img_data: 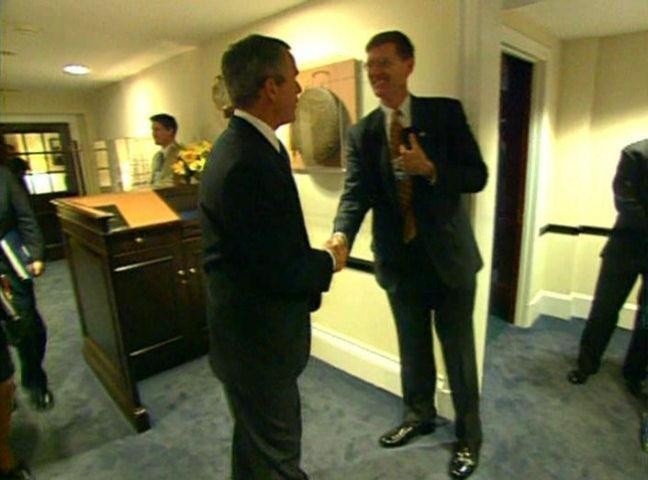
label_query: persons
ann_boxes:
[567,138,648,398]
[329,30,488,479]
[149,113,186,191]
[0,157,53,479]
[200,34,348,480]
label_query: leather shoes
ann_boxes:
[449,448,479,480]
[381,425,435,448]
[632,379,647,401]
[31,390,53,411]
[569,364,586,384]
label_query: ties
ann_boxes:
[389,110,417,244]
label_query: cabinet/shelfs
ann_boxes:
[50,187,210,434]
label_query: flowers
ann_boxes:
[171,137,213,181]
